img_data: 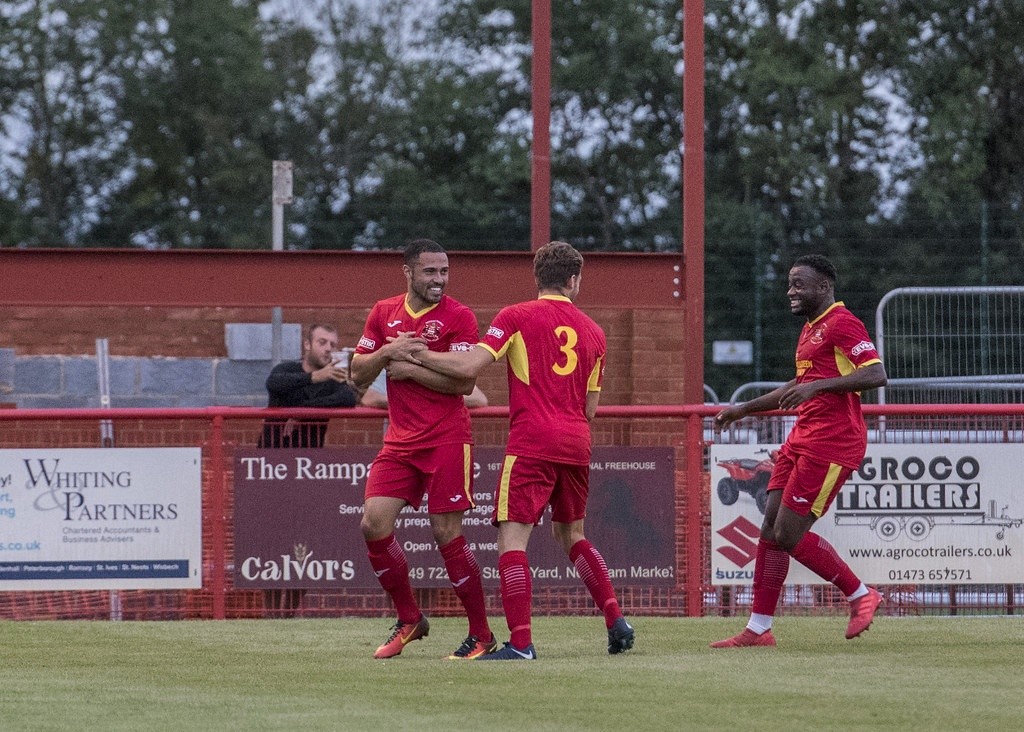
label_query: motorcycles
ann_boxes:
[715,448,784,514]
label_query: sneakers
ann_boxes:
[475,642,536,660]
[444,632,497,660]
[374,611,430,658]
[607,617,636,654]
[709,628,776,647]
[846,586,884,639]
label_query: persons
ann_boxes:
[707,254,888,649]
[257,320,359,616]
[363,368,487,619]
[350,239,501,661]
[384,239,636,661]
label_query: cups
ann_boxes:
[342,348,356,379]
[331,352,349,379]
